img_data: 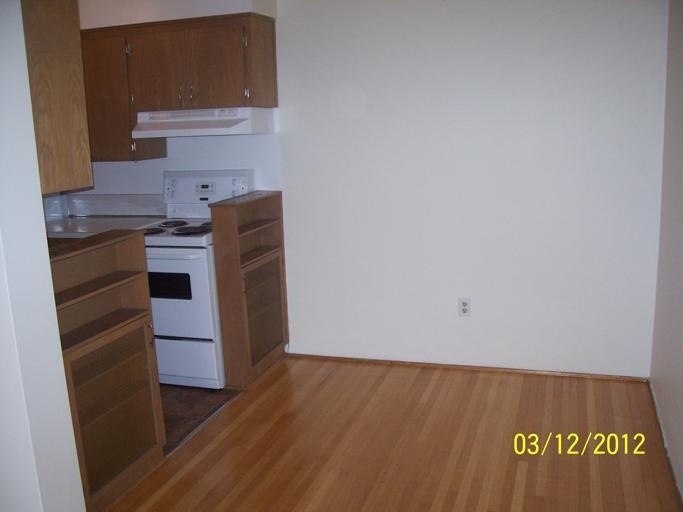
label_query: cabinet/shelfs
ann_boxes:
[125,13,278,112]
[49,228,166,511]
[208,191,289,389]
[19,1,96,199]
[80,26,167,163]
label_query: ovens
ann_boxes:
[145,245,216,341]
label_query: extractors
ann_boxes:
[131,107,272,140]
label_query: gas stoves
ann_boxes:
[130,218,212,243]
